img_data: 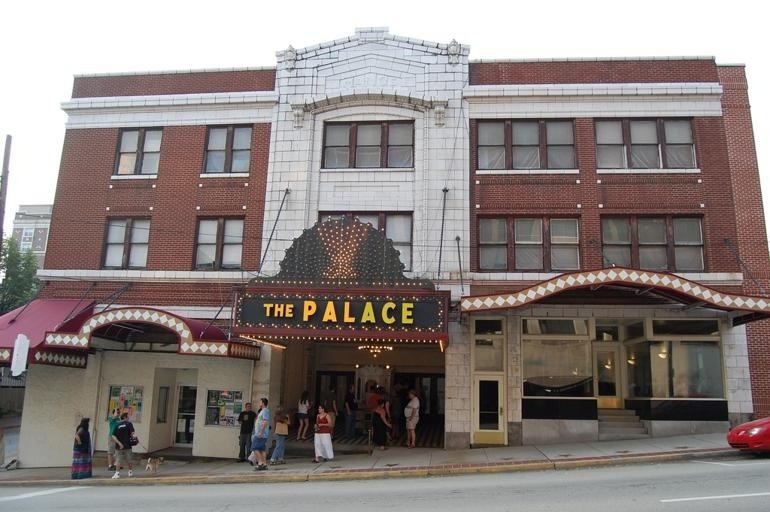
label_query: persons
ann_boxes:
[237,379,422,471]
[107,408,124,470]
[111,412,136,479]
[70,417,92,478]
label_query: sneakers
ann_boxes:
[108,465,132,479]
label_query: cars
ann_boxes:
[650,393,729,419]
[727,416,770,457]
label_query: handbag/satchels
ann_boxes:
[275,422,288,436]
[130,436,138,446]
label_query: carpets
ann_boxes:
[285,409,445,449]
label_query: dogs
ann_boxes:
[144,456,164,472]
